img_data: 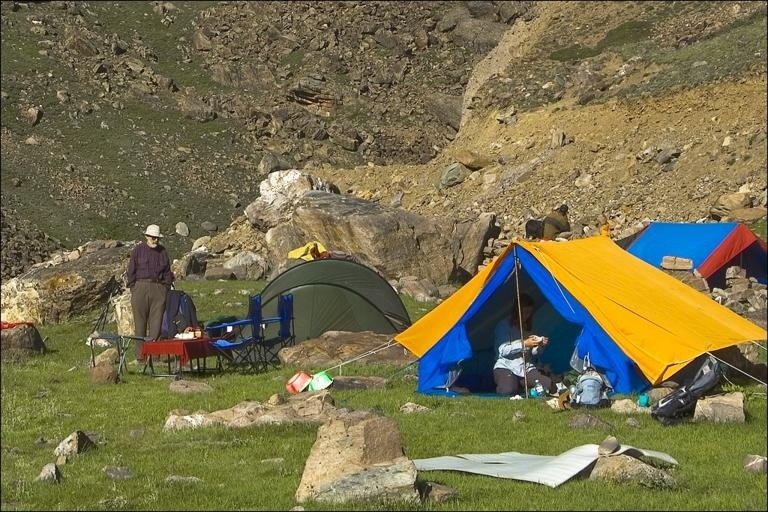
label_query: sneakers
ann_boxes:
[136,358,164,367]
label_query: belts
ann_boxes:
[134,278,164,284]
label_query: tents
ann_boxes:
[228,258,412,368]
[394,235,767,417]
[611,221,768,291]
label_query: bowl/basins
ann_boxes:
[306,372,335,392]
[285,371,314,395]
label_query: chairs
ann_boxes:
[88,290,296,380]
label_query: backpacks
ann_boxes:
[571,367,610,408]
[648,389,697,423]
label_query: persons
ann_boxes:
[541,203,571,241]
[492,292,551,397]
[126,223,172,368]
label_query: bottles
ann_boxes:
[534,379,546,398]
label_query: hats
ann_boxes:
[142,224,165,239]
